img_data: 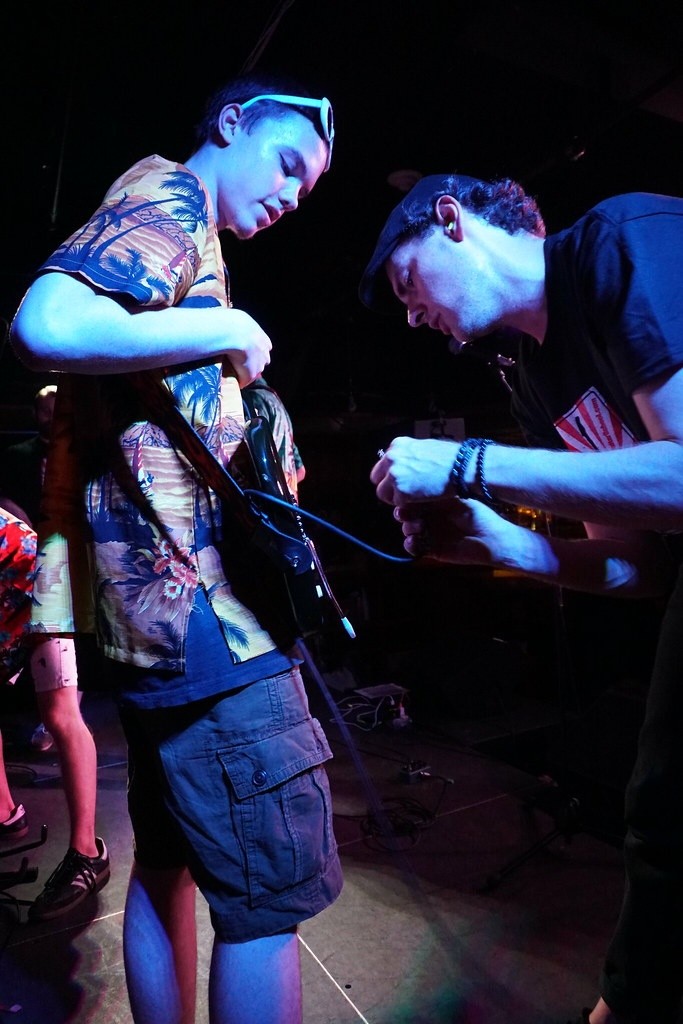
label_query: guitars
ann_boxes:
[215,254,358,654]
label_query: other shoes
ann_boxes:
[32,725,53,750]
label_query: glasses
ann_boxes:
[240,94,336,174]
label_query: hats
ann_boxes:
[360,173,482,312]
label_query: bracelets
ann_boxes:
[449,437,497,505]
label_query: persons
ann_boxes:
[357,172,682,1023]
[0,77,345,1024]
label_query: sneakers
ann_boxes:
[28,835,110,920]
[0,804,30,839]
